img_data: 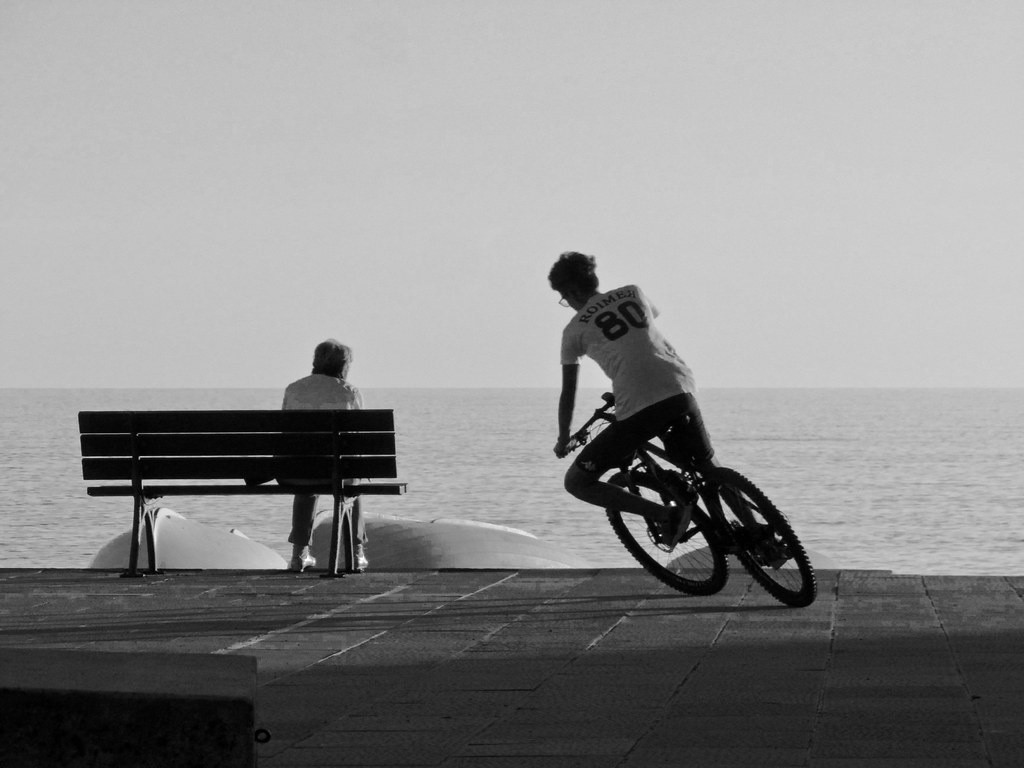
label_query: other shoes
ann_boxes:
[354,554,369,569]
[291,554,316,572]
[663,506,691,547]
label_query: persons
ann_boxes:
[548,251,792,570]
[275,338,371,573]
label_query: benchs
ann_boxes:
[77,408,409,581]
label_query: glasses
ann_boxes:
[559,294,569,307]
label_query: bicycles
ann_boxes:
[564,391,818,609]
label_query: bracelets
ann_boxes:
[558,434,571,447]
